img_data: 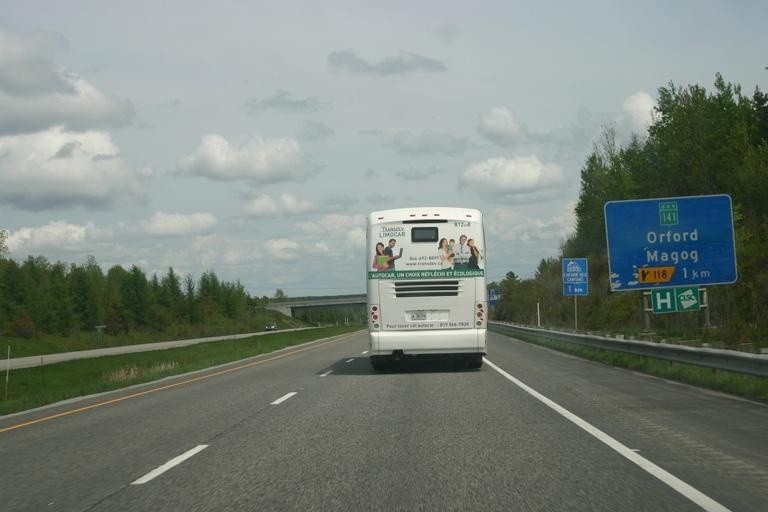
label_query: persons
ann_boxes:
[384,239,403,269]
[372,241,389,271]
[437,235,480,270]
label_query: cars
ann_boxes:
[265,322,277,330]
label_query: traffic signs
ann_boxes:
[607,194,735,288]
[563,258,587,296]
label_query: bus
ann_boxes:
[362,205,490,369]
